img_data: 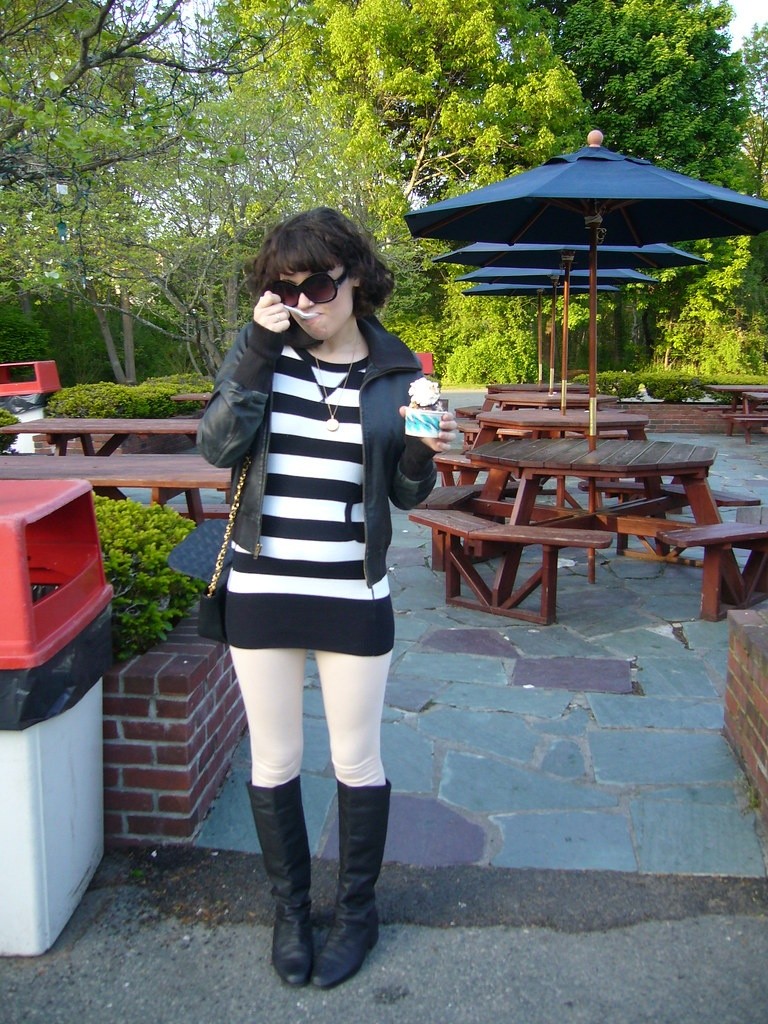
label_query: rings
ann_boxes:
[275,314,280,323]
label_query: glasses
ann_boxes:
[264,263,349,308]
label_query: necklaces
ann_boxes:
[315,328,359,431]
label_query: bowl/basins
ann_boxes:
[405,407,446,438]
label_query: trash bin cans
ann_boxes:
[0,359,62,454]
[412,353,434,378]
[2,477,114,954]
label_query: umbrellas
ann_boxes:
[404,130,768,452]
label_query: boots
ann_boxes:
[246,775,311,987]
[309,779,392,991]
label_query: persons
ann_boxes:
[196,207,456,989]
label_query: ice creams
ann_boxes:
[404,377,444,439]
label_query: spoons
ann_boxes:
[260,297,319,319]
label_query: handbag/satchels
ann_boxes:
[167,518,232,643]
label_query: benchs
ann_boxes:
[407,404,768,625]
[142,502,231,519]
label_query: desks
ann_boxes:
[473,410,649,432]
[484,392,619,407]
[464,439,745,607]
[171,393,211,408]
[704,385,767,391]
[486,384,593,392]
[0,452,232,526]
[0,418,201,456]
[742,392,768,402]
